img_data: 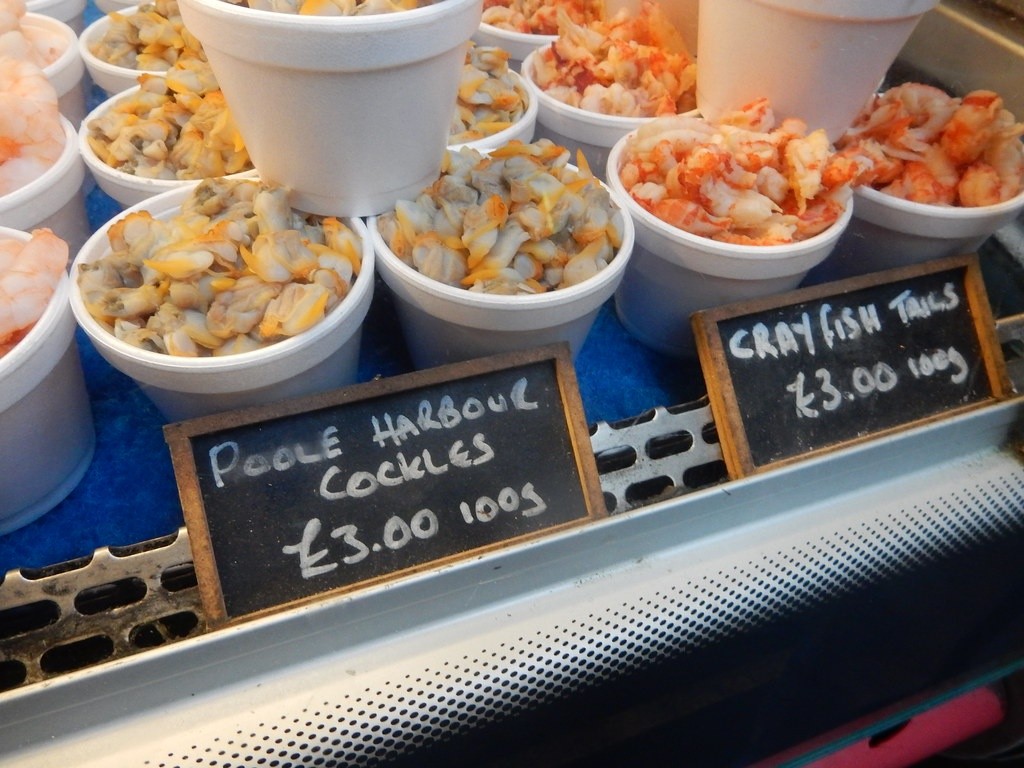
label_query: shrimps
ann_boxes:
[619,96,844,245]
[1,1,69,357]
[823,82,1024,210]
[480,0,697,117]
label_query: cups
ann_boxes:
[0,0,1024,535]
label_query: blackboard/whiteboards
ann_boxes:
[690,254,1016,482]
[162,338,608,633]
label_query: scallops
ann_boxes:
[77,176,363,358]
[89,0,210,73]
[87,59,254,179]
[377,137,622,297]
[221,0,434,17]
[445,39,527,145]
